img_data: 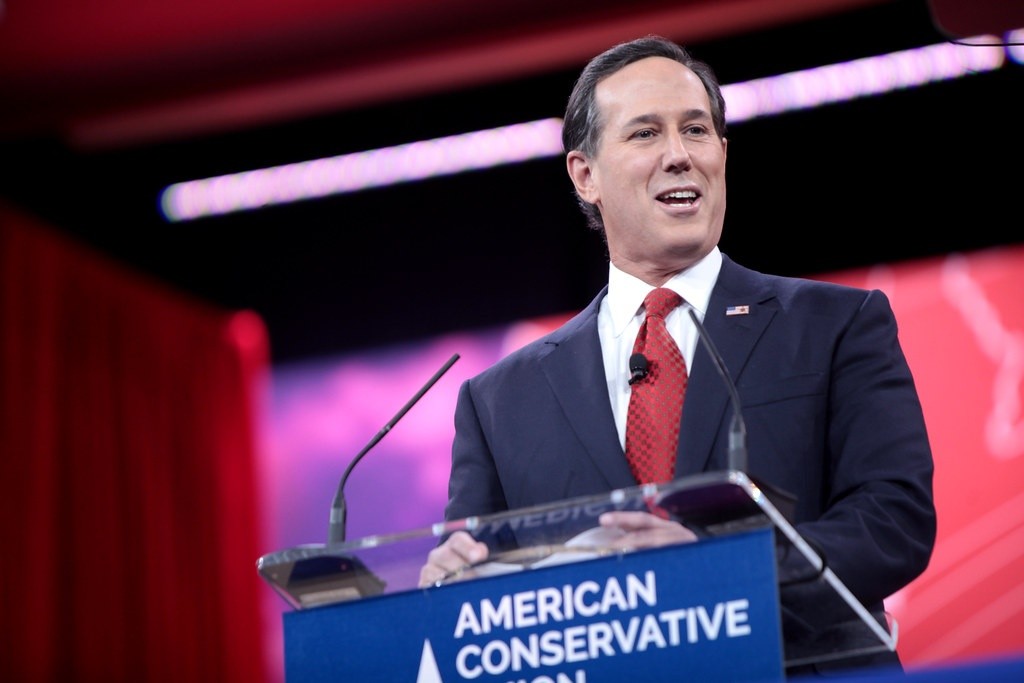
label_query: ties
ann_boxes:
[624,288,689,521]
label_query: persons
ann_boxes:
[417,34,938,679]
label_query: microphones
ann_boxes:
[287,353,461,608]
[630,353,647,379]
[657,309,798,538]
